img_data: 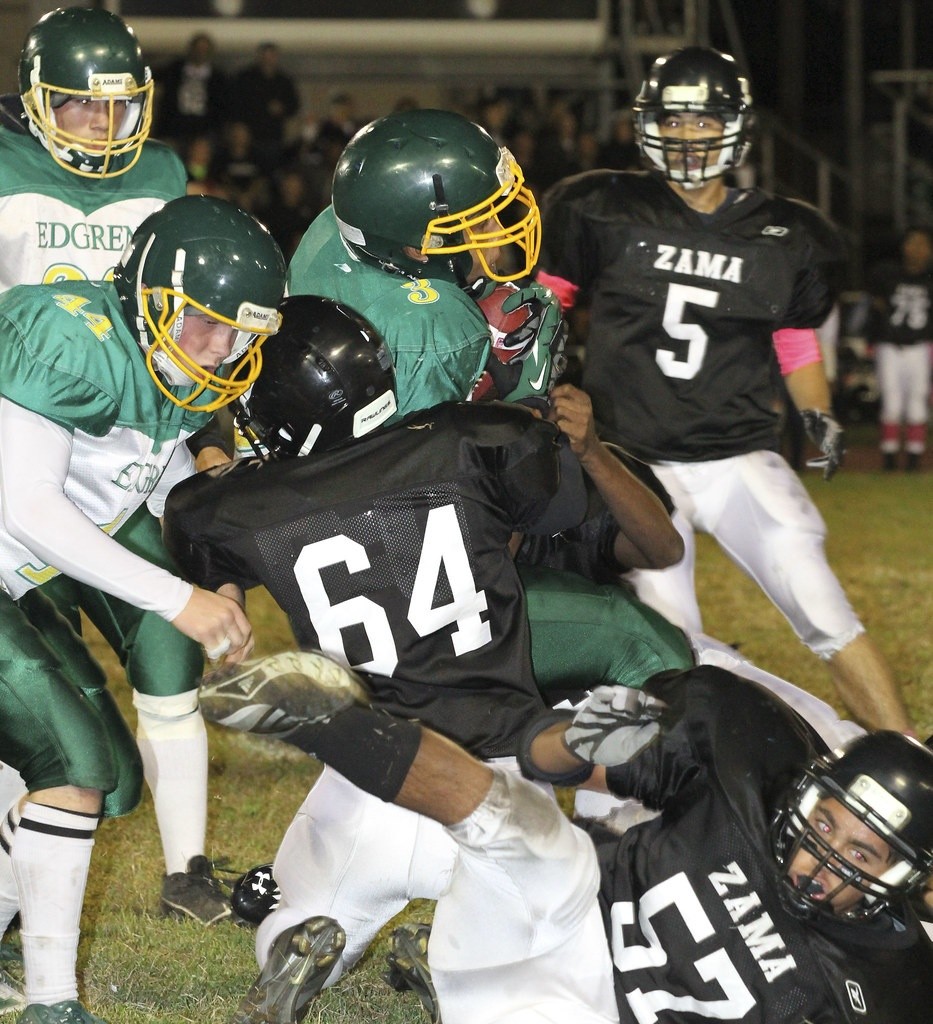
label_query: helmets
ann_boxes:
[228,296,399,457]
[763,727,933,930]
[15,7,157,179]
[112,192,288,412]
[332,109,541,299]
[632,46,758,189]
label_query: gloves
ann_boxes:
[561,685,674,768]
[484,284,569,419]
[802,408,848,482]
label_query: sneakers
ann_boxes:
[229,860,282,923]
[159,854,234,926]
[17,1000,108,1024]
[198,649,391,744]
[383,921,442,1024]
[0,939,28,1002]
[227,914,347,1024]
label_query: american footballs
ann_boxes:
[464,283,536,403]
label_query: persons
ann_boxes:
[0,7,933,1024]
[529,46,915,741]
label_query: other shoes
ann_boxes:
[881,449,896,472]
[906,451,923,473]
